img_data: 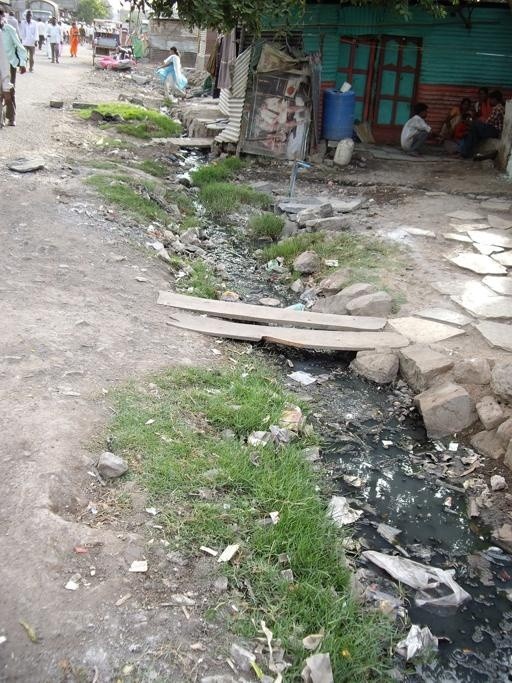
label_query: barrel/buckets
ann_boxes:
[322,89,356,139]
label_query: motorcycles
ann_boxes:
[117,46,134,61]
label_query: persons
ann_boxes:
[37,17,119,49]
[0,5,29,129]
[400,101,433,158]
[19,8,39,73]
[440,86,505,156]
[157,46,189,102]
[47,16,61,63]
[69,22,79,57]
